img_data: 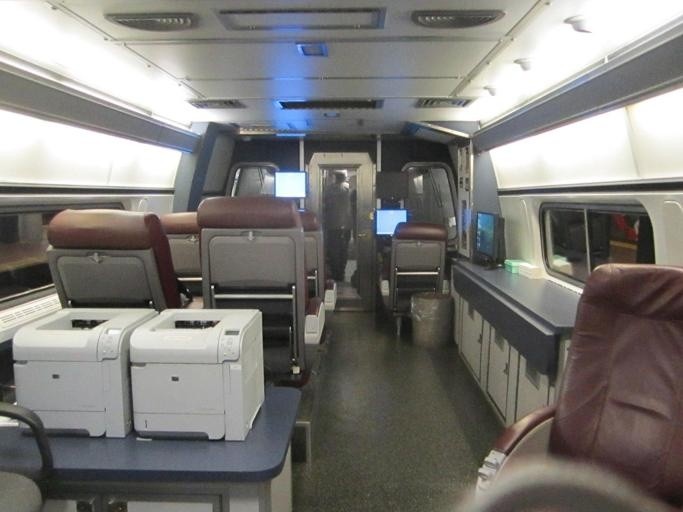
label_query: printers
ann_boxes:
[127,307,266,442]
[11,307,160,438]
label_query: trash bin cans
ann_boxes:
[411,291,453,350]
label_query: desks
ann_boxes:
[0,386,303,511]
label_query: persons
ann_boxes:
[324,169,358,282]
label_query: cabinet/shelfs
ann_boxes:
[450,258,582,429]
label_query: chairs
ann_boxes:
[161,211,202,291]
[0,404,53,512]
[476,262,683,512]
[379,222,447,335]
[46,208,181,309]
[198,196,306,371]
[298,211,325,301]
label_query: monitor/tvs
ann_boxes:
[474,209,506,270]
[374,208,409,237]
[274,171,308,198]
